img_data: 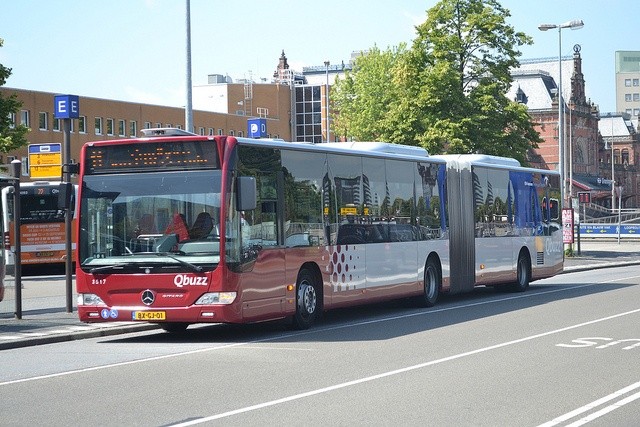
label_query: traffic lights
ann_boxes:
[577,192,591,205]
[542,196,560,222]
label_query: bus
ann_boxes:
[0,180,79,276]
[57,127,564,333]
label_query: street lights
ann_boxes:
[538,19,584,211]
